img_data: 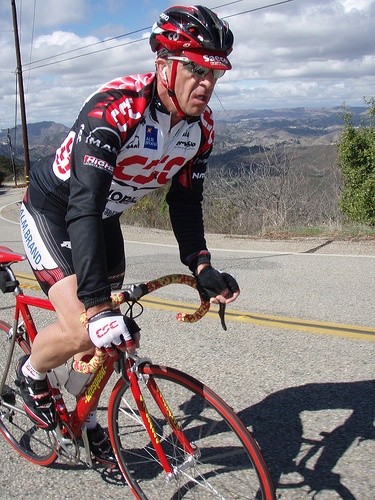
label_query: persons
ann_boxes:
[15,4,240,464]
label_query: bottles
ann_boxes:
[64,354,97,398]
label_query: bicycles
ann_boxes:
[0,245,277,500]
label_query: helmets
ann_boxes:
[149,4,234,70]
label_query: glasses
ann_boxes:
[165,56,226,78]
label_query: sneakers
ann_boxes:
[73,423,117,463]
[15,354,58,430]
